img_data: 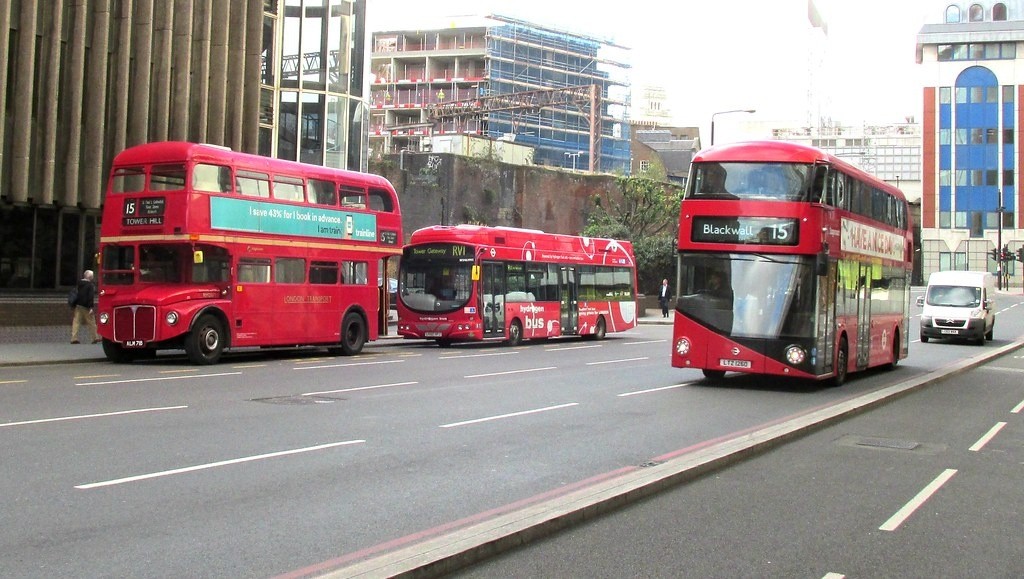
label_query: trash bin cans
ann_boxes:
[638,293,646,317]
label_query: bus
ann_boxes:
[96,142,402,366]
[671,141,913,386]
[396,224,639,348]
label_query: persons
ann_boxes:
[70,270,102,344]
[385,92,391,104]
[439,89,445,103]
[657,278,672,318]
[706,273,723,289]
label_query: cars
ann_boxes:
[378,276,403,304]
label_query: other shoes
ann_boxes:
[71,340,80,344]
[92,337,102,344]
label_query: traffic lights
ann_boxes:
[1016,247,1024,263]
[990,248,997,261]
[1000,247,1015,261]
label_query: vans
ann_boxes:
[915,270,996,345]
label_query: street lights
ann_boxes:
[563,150,584,170]
[710,108,757,146]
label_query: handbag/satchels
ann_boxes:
[68,285,78,306]
